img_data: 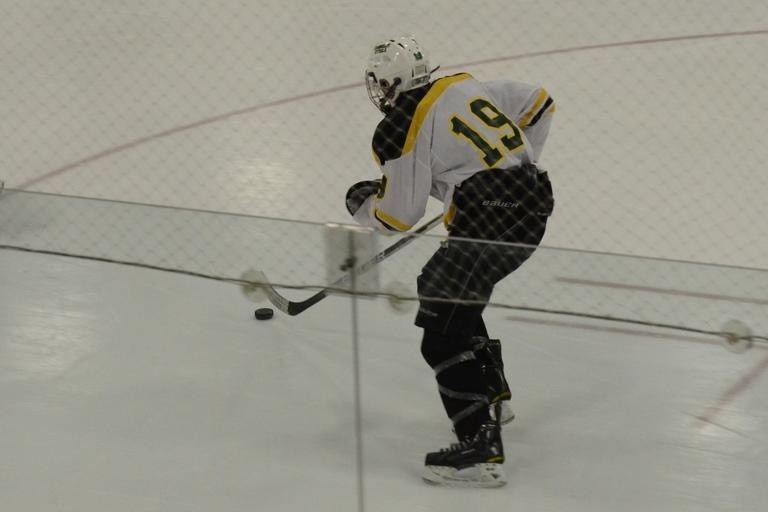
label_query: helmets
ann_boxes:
[364,37,432,94]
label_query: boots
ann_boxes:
[463,335,514,403]
[423,422,505,468]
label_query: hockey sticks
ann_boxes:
[260,213,445,316]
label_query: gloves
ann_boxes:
[344,176,383,217]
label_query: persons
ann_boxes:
[345,34,556,473]
